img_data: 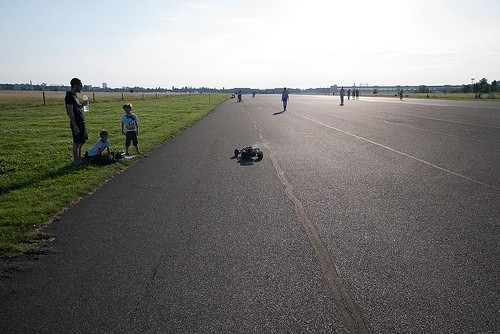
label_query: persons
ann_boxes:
[235,91,237,95]
[340,86,360,106]
[237,90,242,102]
[121,103,143,156]
[84,130,110,163]
[252,92,255,98]
[399,89,404,99]
[281,87,289,111]
[65,78,89,166]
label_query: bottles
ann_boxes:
[82,95,90,112]
